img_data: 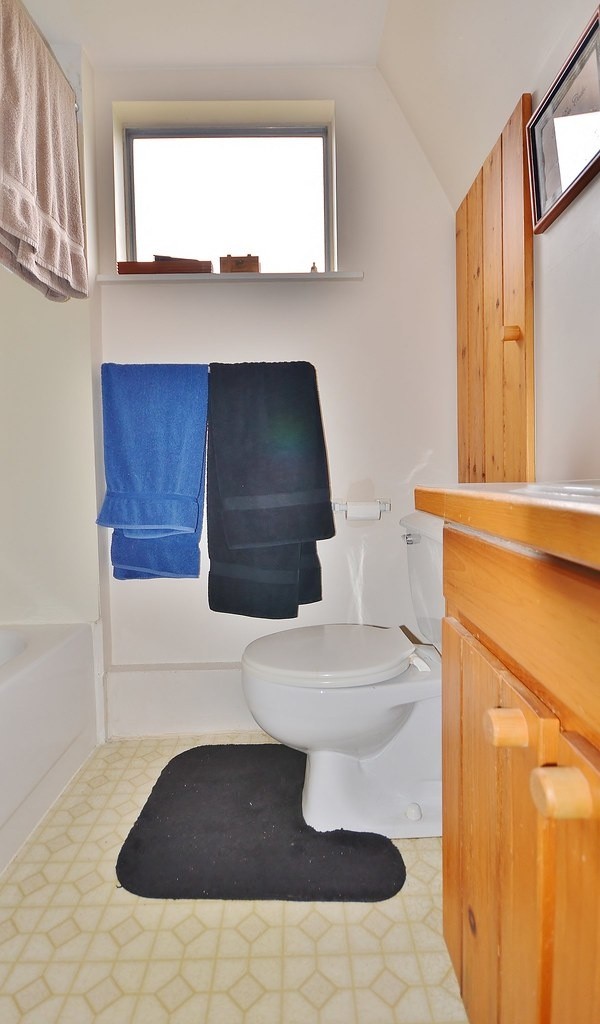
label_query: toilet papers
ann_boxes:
[345,500,381,521]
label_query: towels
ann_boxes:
[208,361,341,620]
[95,362,209,582]
[1,0,90,303]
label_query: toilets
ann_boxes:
[241,510,443,839]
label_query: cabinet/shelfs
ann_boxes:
[413,3,600,1024]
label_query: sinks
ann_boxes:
[507,478,600,503]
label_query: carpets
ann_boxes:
[115,743,407,904]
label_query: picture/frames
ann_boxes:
[525,6,600,235]
